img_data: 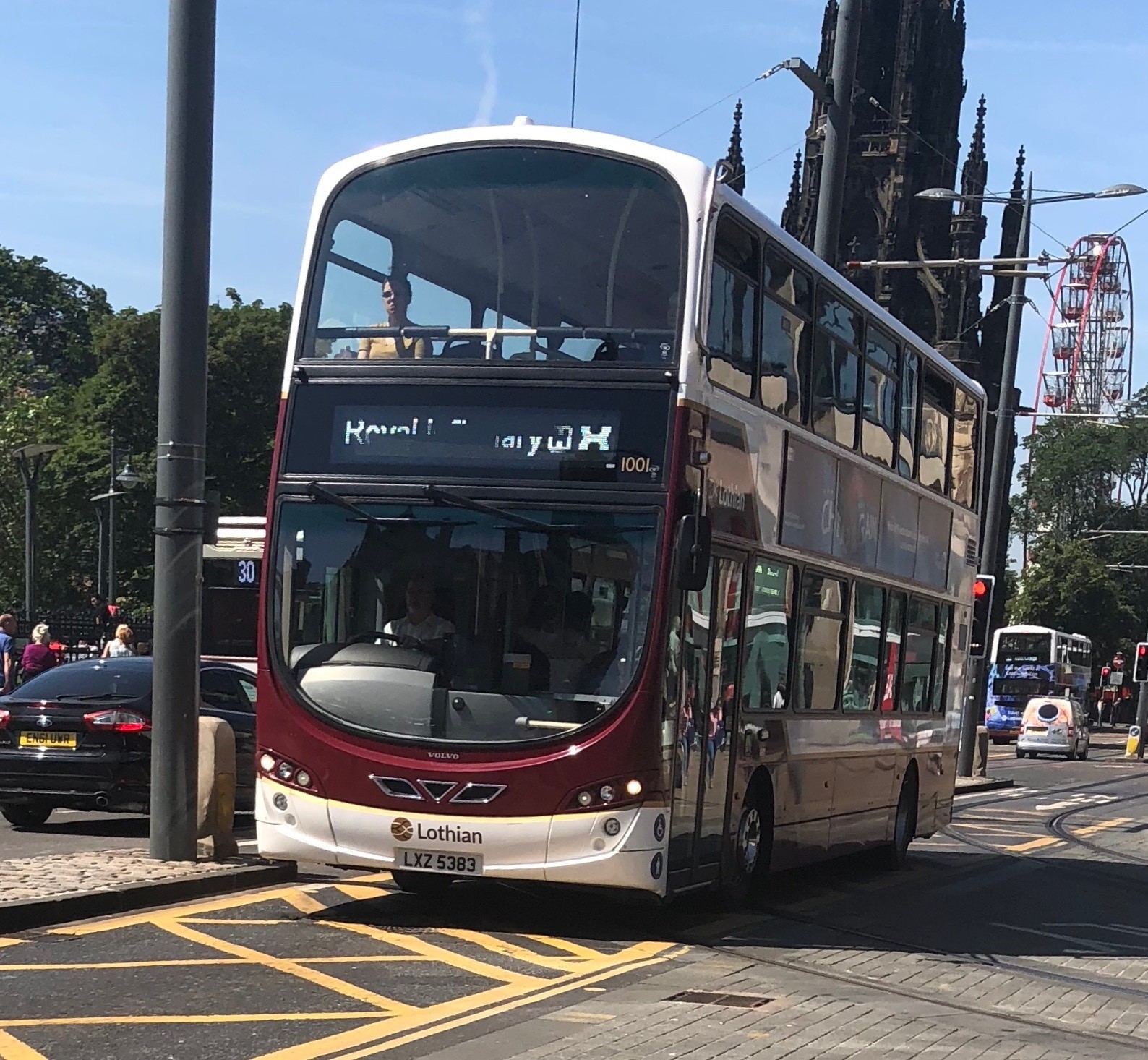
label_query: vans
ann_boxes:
[1015,698,1093,760]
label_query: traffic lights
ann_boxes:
[1100,667,1111,686]
[969,574,996,659]
[1132,642,1148,682]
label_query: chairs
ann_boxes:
[440,336,502,360]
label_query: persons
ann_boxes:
[88,590,121,621]
[0,614,17,698]
[98,624,138,659]
[372,570,457,669]
[18,621,57,685]
[705,694,730,791]
[356,273,438,361]
[771,682,786,710]
[675,696,695,783]
[669,612,681,715]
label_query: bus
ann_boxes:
[254,117,987,910]
[985,624,1092,744]
[201,516,351,711]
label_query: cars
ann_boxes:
[0,657,260,827]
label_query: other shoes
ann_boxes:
[709,781,713,788]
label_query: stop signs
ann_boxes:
[1112,655,1125,668]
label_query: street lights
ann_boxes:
[109,445,146,606]
[914,184,1148,779]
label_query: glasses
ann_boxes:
[383,291,408,298]
